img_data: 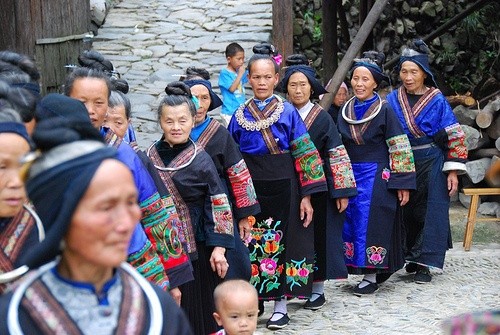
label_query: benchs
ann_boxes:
[462,188,500,252]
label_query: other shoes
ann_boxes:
[353,279,380,296]
[255,304,290,328]
[304,292,327,310]
[406,261,432,284]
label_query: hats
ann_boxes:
[349,62,392,86]
[22,147,118,269]
[183,80,223,112]
[282,66,330,95]
[318,80,349,100]
[398,53,439,90]
[0,122,32,150]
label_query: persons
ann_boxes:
[60,50,195,309]
[209,278,260,335]
[0,50,139,147]
[386,39,469,286]
[177,63,261,284]
[100,75,194,274]
[217,42,251,128]
[0,82,46,298]
[29,118,170,296]
[224,42,329,329]
[318,78,350,125]
[0,118,195,335]
[337,49,419,297]
[274,53,358,310]
[142,81,238,335]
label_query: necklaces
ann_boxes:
[341,90,382,124]
[1,204,49,286]
[7,253,164,335]
[147,135,196,172]
[233,94,287,131]
